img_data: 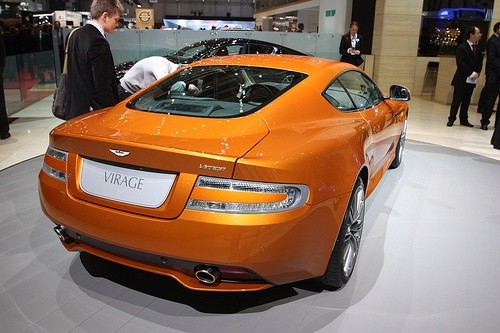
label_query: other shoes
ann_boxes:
[481,125,487,130]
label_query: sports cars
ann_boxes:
[38,54,410,293]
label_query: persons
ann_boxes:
[0,40,12,140]
[480,30,500,131]
[52,0,124,130]
[116,56,186,103]
[297,23,305,33]
[477,22,500,114]
[447,27,481,127]
[338,22,365,67]
[0,20,65,81]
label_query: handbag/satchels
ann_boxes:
[52,72,90,119]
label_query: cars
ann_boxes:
[114,38,314,81]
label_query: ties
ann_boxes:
[472,44,477,57]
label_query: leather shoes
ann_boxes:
[447,120,454,126]
[460,120,474,127]
